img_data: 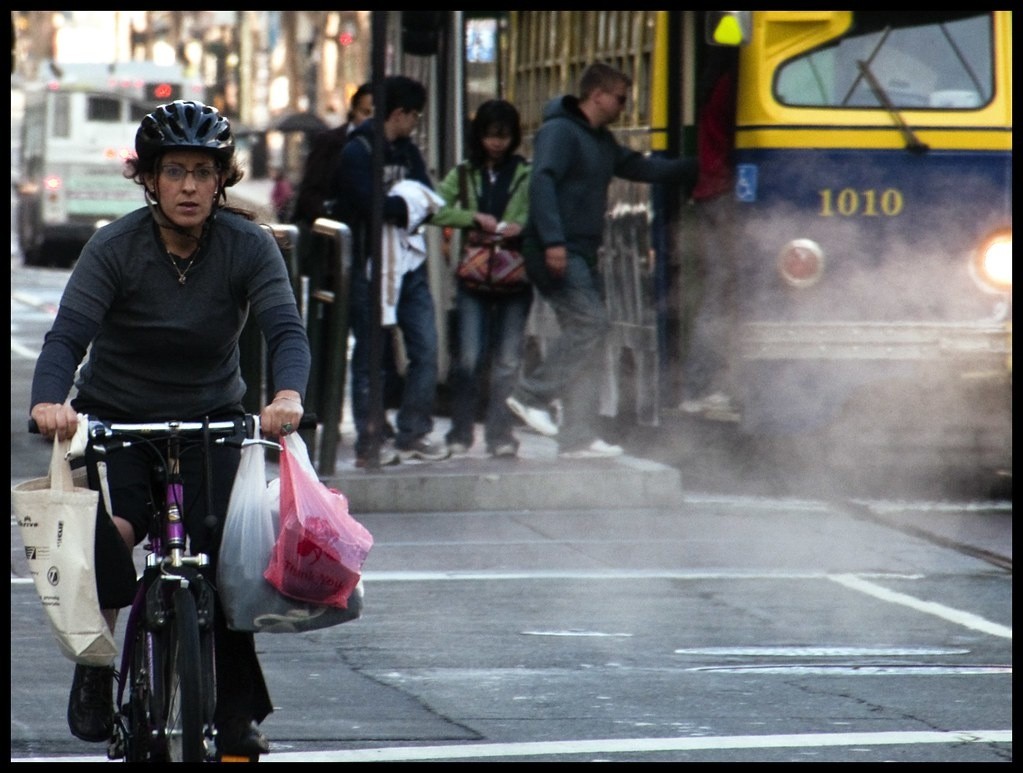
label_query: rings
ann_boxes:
[282,421,294,433]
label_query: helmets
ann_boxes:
[135,99,236,159]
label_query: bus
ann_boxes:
[13,60,199,268]
[350,9,1013,491]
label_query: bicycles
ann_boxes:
[28,410,318,763]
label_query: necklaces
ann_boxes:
[165,244,201,283]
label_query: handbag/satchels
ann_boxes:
[12,413,120,662]
[455,230,533,298]
[215,419,375,633]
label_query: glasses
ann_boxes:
[157,163,220,182]
[603,89,627,106]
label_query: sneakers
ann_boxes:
[355,438,452,466]
[66,661,114,742]
[216,715,270,754]
[560,439,625,459]
[506,396,560,436]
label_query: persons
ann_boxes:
[29,99,309,754]
[272,74,535,472]
[507,61,683,460]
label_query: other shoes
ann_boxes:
[447,441,515,461]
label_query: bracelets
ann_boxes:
[273,396,302,405]
